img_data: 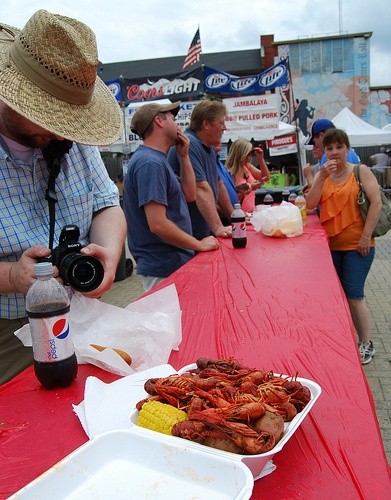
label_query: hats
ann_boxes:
[1,9,124,146]
[307,118,334,145]
[130,99,181,137]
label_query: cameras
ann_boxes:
[36,224,105,293]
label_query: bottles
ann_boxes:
[282,187,290,201]
[230,203,247,249]
[288,189,297,204]
[23,262,78,390]
[295,191,307,226]
[263,191,274,206]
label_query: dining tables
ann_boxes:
[0,208,391,500]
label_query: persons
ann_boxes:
[370,147,390,187]
[303,118,360,185]
[0,9,128,389]
[123,100,220,292]
[225,138,270,212]
[215,142,252,207]
[305,129,383,363]
[115,174,125,197]
[166,98,234,240]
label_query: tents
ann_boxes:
[278,107,391,167]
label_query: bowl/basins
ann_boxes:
[6,361,323,500]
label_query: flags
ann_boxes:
[183,29,202,71]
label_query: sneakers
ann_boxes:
[357,340,376,364]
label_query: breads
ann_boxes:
[91,344,131,366]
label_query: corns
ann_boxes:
[137,399,189,436]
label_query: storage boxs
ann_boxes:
[254,186,304,204]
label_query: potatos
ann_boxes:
[201,410,284,456]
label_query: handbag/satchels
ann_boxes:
[251,198,303,237]
[353,164,391,236]
